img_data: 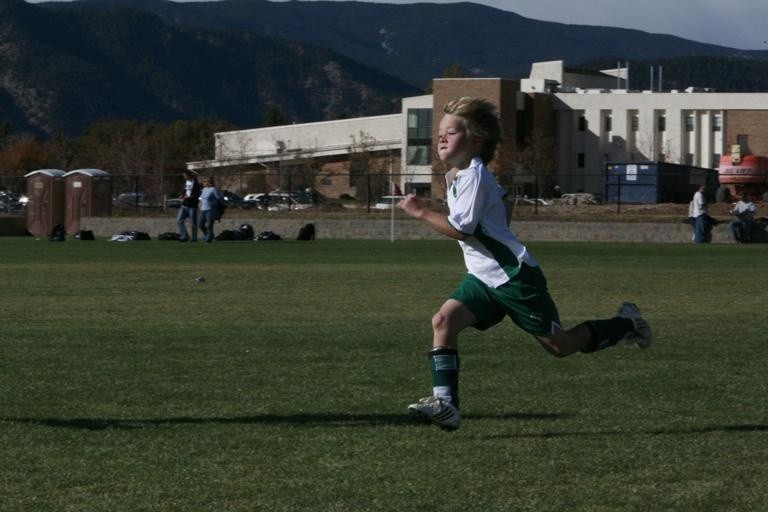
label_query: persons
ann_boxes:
[196,176,221,244]
[728,190,757,245]
[691,184,707,243]
[174,169,201,243]
[393,95,652,433]
[687,198,704,242]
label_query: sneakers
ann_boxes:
[618,302,651,350]
[407,394,460,433]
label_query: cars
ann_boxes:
[515,193,596,206]
[0,191,28,211]
[111,190,316,211]
[376,196,406,210]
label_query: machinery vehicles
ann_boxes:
[715,145,768,204]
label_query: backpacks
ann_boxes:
[48,224,180,242]
[216,223,315,242]
